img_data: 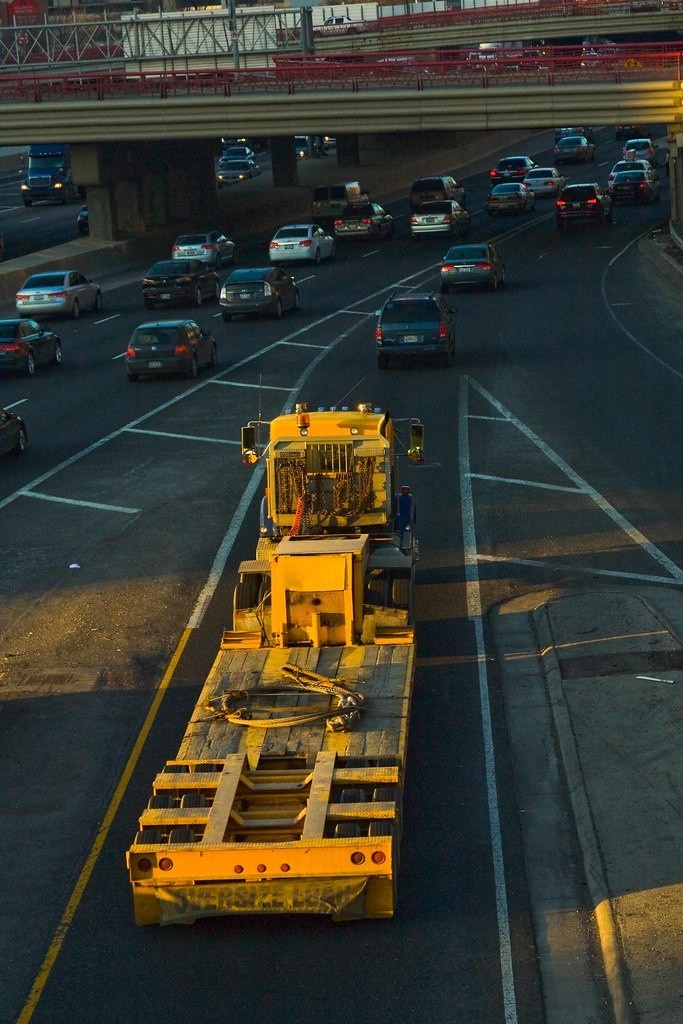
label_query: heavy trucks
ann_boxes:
[125,404,425,922]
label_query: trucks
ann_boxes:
[18,142,87,206]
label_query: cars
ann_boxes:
[486,155,565,216]
[377,42,621,71]
[312,15,368,37]
[607,149,661,203]
[219,266,299,322]
[170,229,237,267]
[554,126,660,166]
[15,271,103,320]
[269,223,337,265]
[334,201,395,243]
[78,208,91,235]
[440,243,507,291]
[0,408,27,455]
[124,318,217,383]
[0,318,63,376]
[411,201,471,240]
[218,137,335,187]
[142,260,221,312]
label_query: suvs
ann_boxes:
[373,292,459,371]
[408,175,467,209]
[309,182,371,222]
[555,183,614,227]
